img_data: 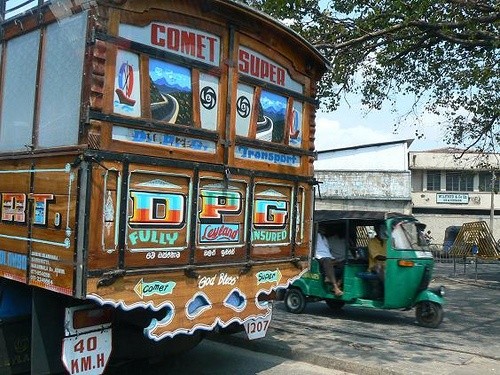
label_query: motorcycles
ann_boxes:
[275,207,446,328]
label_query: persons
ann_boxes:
[315,224,344,296]
[368,223,387,280]
[425,230,435,243]
[328,224,346,285]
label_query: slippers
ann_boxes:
[333,289,343,296]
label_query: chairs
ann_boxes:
[355,245,383,296]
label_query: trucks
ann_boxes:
[0,1,328,375]
